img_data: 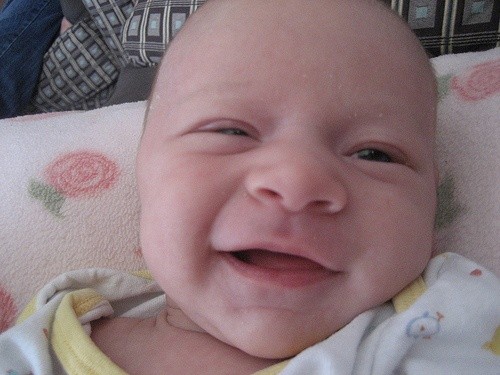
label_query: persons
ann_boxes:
[0,1,499,374]
[0,0,88,119]
